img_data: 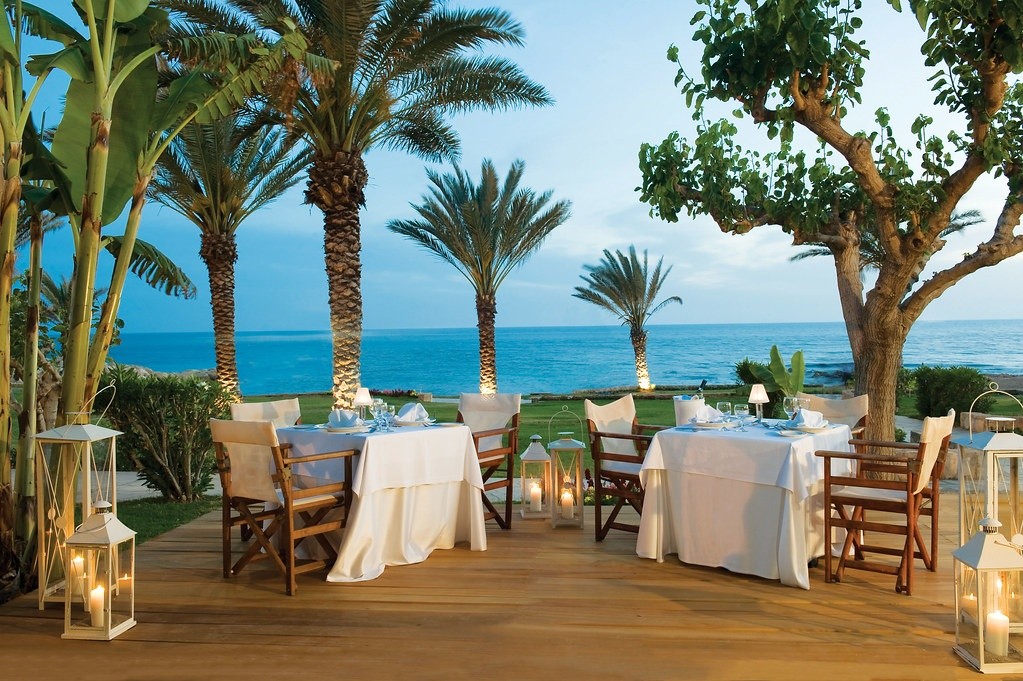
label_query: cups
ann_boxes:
[793,398,810,413]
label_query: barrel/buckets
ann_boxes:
[672,395,705,426]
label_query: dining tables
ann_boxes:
[636,416,862,594]
[258,423,488,582]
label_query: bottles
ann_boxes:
[690,380,707,400]
[396,418,437,427]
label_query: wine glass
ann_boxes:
[381,405,396,432]
[368,398,383,433]
[783,396,798,421]
[716,402,731,432]
[734,404,749,432]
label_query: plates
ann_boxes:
[776,430,808,438]
[324,426,371,433]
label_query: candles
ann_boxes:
[72,557,85,595]
[529,485,542,512]
[985,610,1011,656]
[560,491,575,518]
[997,580,1003,593]
[116,572,131,589]
[89,586,104,627]
[962,594,977,607]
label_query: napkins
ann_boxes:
[328,409,359,426]
[787,408,824,426]
[688,404,729,423]
[396,402,430,422]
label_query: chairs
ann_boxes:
[812,407,956,596]
[229,397,303,428]
[584,392,673,544]
[795,390,869,537]
[208,417,361,595]
[458,391,522,530]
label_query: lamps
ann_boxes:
[353,387,374,419]
[748,384,772,430]
